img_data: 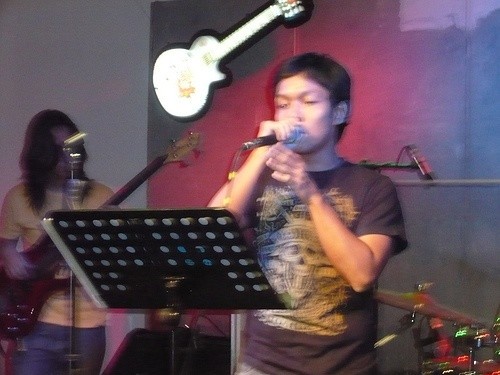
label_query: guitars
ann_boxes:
[0,131,202,339]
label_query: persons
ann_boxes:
[0,108,124,375]
[203,51,408,375]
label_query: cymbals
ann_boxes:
[375,290,475,324]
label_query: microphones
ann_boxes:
[417,284,454,358]
[242,125,305,151]
[406,145,434,181]
